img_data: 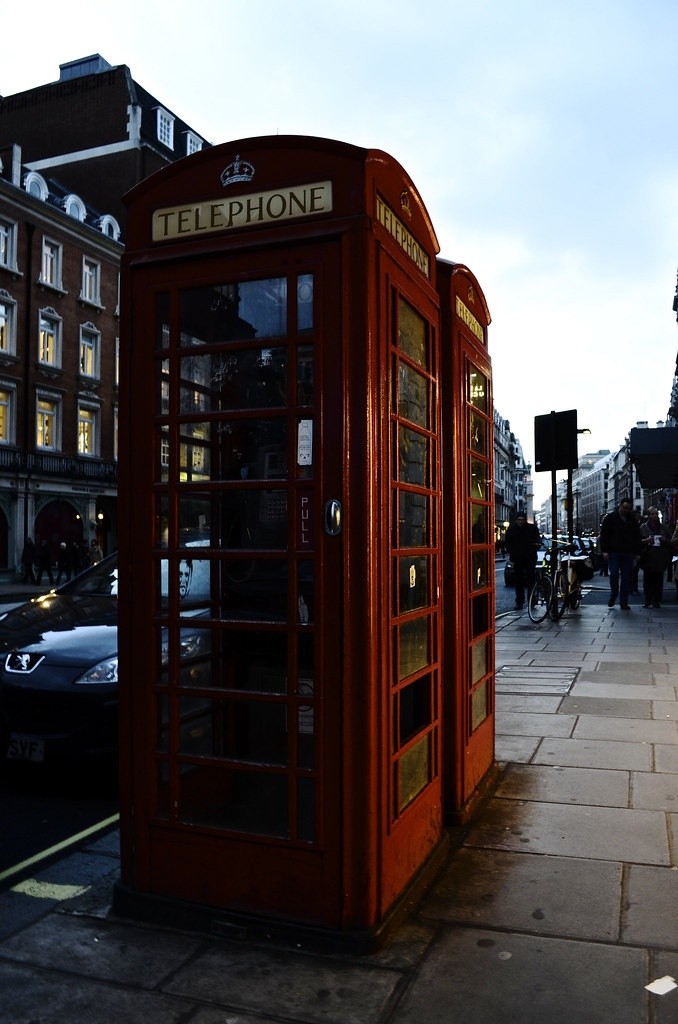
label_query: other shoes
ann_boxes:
[608,596,617,607]
[514,606,523,610]
[621,604,632,610]
[653,603,661,609]
[642,600,652,608]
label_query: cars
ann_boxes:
[504,534,599,589]
[0,528,219,770]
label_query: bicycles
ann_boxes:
[528,536,588,625]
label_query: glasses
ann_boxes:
[622,504,631,511]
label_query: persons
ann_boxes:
[640,506,672,608]
[504,511,541,610]
[600,498,641,610]
[629,505,678,599]
[21,537,104,586]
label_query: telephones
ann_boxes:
[226,442,316,546]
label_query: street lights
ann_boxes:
[573,488,583,533]
